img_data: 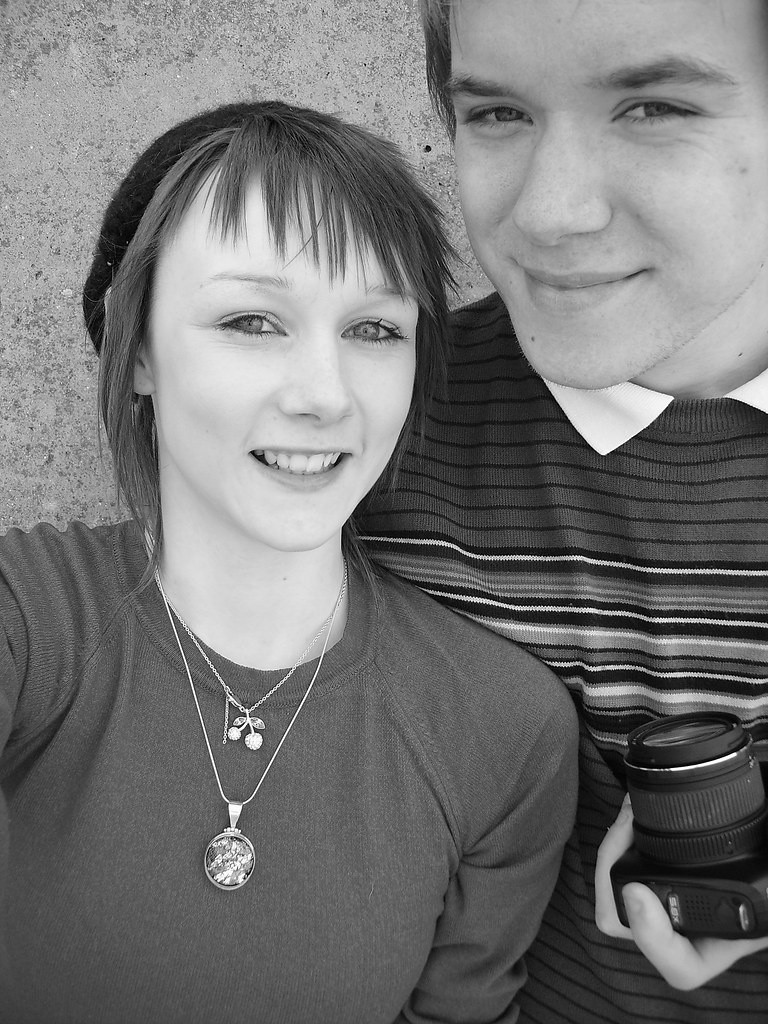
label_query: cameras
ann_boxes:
[610,710,768,940]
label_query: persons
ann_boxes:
[346,0,768,1024]
[1,102,576,1024]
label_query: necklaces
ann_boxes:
[146,528,347,893]
